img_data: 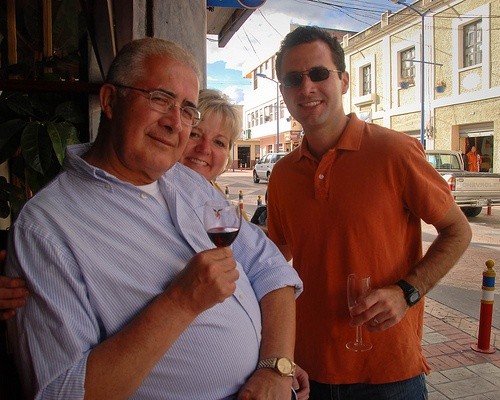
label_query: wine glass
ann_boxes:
[202,201,242,251]
[345,273,374,352]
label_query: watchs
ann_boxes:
[256,357,296,377]
[395,279,421,308]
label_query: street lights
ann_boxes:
[255,73,281,153]
[389,0,432,144]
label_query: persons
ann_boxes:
[466,146,482,173]
[0,37,309,400]
[267,25,473,400]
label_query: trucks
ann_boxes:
[424,149,500,217]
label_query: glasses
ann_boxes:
[279,66,342,88]
[112,84,204,127]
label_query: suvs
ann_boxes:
[253,152,288,183]
